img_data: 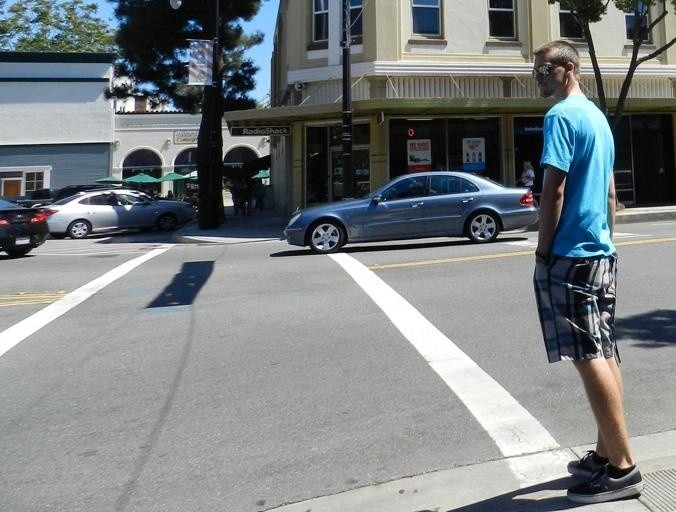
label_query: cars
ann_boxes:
[0,183,196,242]
[0,197,50,260]
[277,167,540,257]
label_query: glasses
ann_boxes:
[531,64,563,79]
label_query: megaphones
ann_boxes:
[294,80,307,91]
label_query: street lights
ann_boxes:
[166,0,224,234]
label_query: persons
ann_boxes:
[531,40,644,505]
[230,180,267,217]
[514,161,535,191]
[152,187,200,212]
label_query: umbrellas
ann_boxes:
[159,172,189,198]
[251,171,270,185]
[121,173,163,191]
[95,175,120,189]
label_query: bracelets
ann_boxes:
[534,250,550,260]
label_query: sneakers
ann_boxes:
[567,450,610,475]
[566,464,644,503]
